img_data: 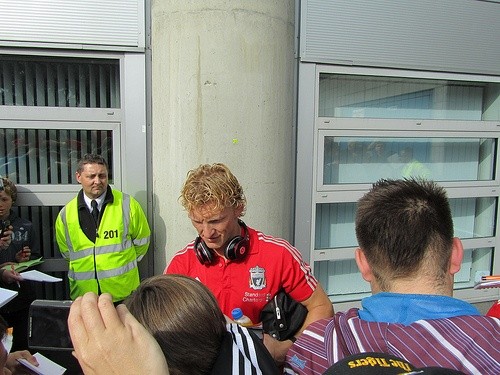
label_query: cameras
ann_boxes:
[27,299,75,351]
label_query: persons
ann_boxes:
[66,277,232,375]
[324,136,434,180]
[286,182,500,375]
[55,154,149,303]
[0,177,38,375]
[163,162,334,363]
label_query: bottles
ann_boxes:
[231,308,253,326]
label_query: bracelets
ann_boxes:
[289,336,297,343]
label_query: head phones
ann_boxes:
[194,219,249,265]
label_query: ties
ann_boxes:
[91,200,99,225]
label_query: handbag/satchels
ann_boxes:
[259,288,307,343]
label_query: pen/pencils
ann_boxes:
[11,264,21,288]
[481,275,500,281]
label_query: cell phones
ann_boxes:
[1,220,10,237]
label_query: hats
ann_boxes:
[322,352,467,375]
